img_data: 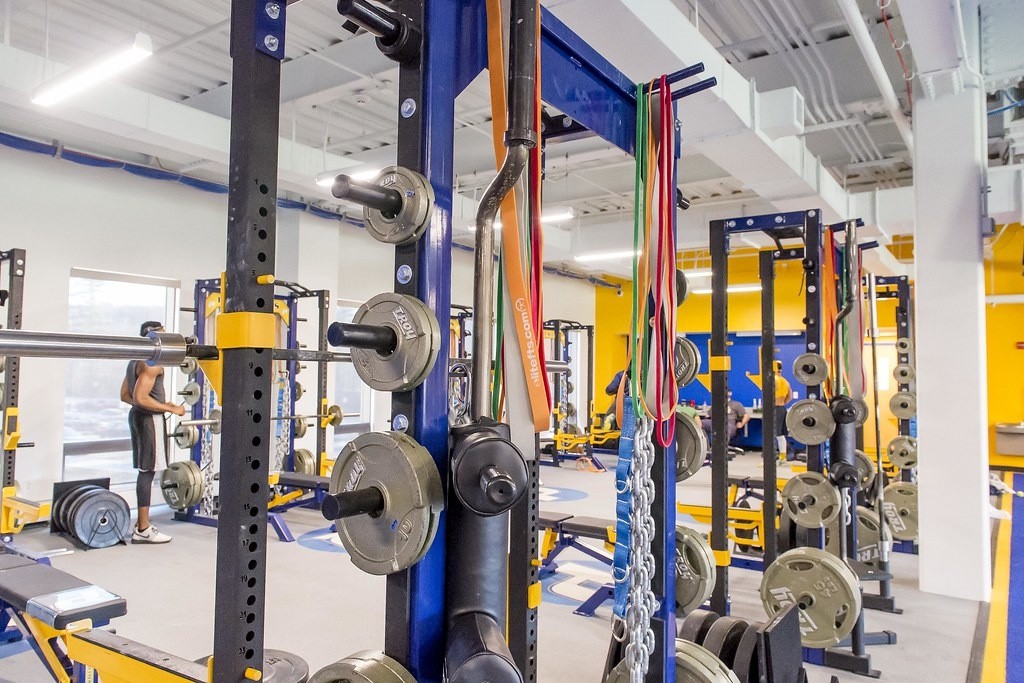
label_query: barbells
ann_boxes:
[865,272,899,597]
[574,430,614,438]
[178,405,362,435]
[0,328,573,374]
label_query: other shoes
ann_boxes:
[758,461,763,468]
[603,413,616,431]
[776,461,789,467]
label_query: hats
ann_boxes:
[728,386,731,391]
[139,322,164,337]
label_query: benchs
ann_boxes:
[538,512,618,578]
[0,553,127,659]
[276,472,330,509]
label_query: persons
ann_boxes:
[757,360,792,467]
[675,384,751,443]
[120,321,185,544]
[605,369,632,428]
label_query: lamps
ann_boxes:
[315,100,382,187]
[469,153,574,231]
[679,205,762,294]
[27,1,152,107]
[571,184,643,263]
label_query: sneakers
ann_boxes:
[132,521,158,535]
[131,525,173,544]
